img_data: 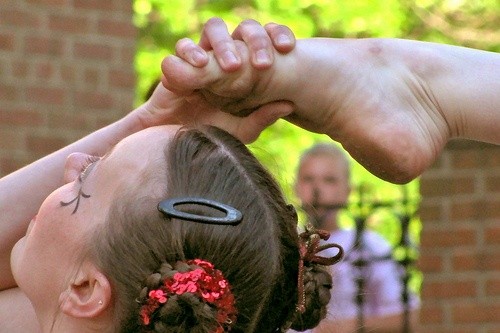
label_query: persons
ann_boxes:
[1,16,499,333]
[291,144,421,332]
